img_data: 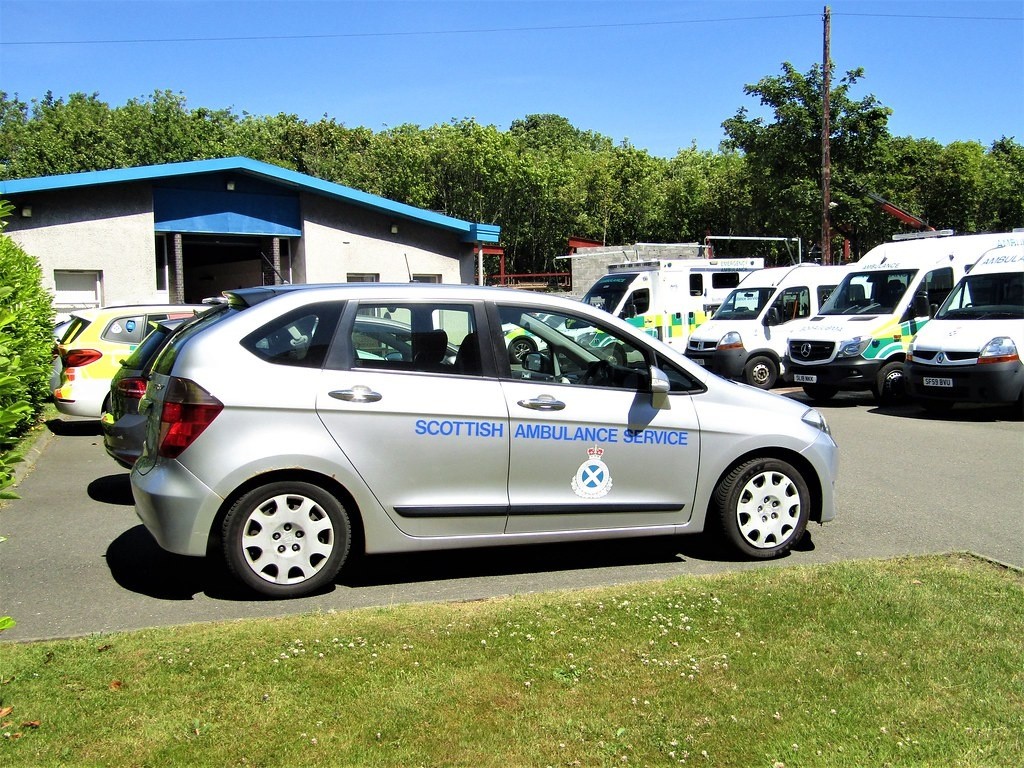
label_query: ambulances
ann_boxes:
[783,229,1024,406]
[681,261,859,390]
[547,257,764,369]
[907,245,1024,407]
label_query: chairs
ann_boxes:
[455,334,484,374]
[882,279,902,304]
[414,328,448,364]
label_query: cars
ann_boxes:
[127,282,840,602]
[500,311,583,366]
[49,295,463,471]
